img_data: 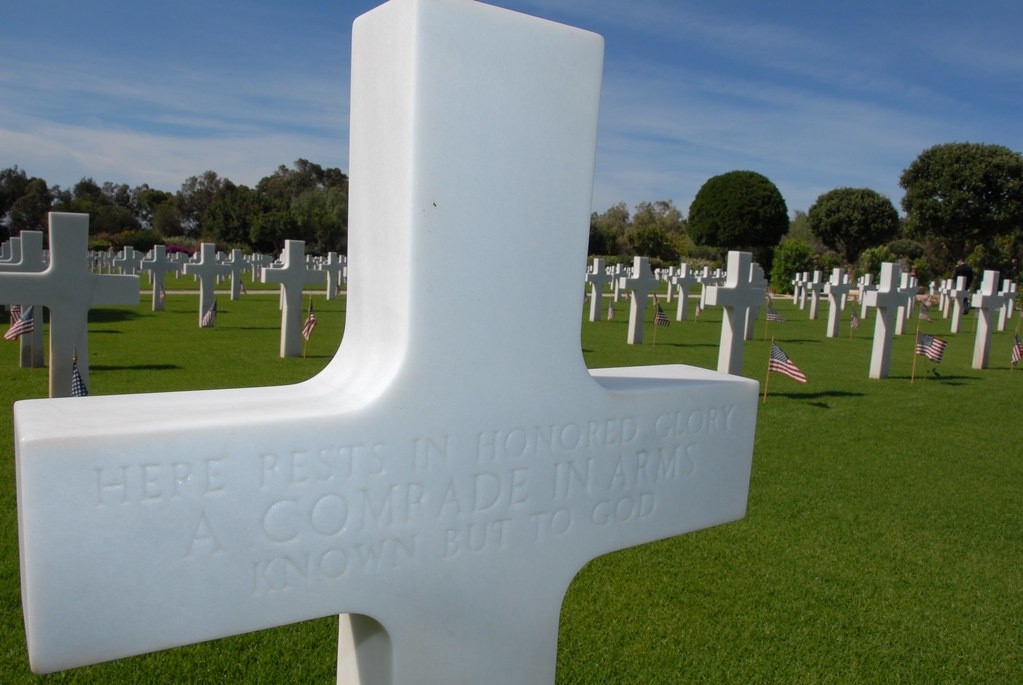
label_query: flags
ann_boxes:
[1,297,1023,402]
